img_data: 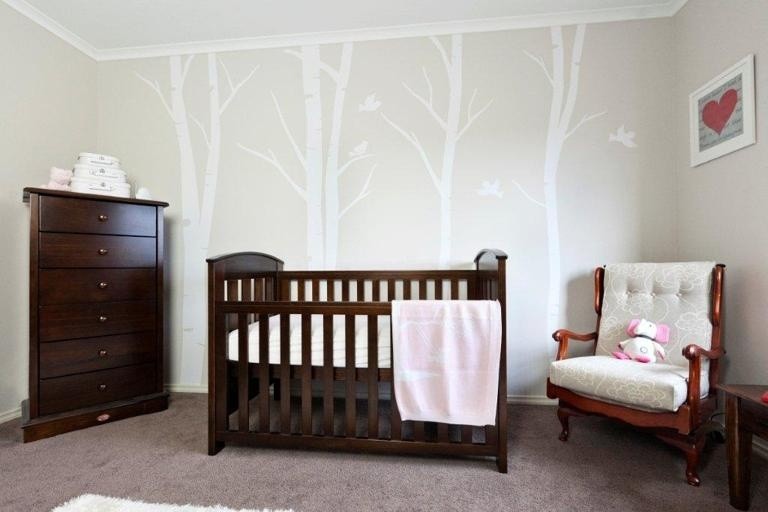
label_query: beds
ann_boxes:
[206,247,508,473]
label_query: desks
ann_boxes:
[715,383,768,512]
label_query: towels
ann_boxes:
[390,298,502,427]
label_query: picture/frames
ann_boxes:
[689,51,756,169]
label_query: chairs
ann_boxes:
[547,261,724,486]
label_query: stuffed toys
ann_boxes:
[612,317,670,364]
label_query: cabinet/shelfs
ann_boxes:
[19,186,170,443]
